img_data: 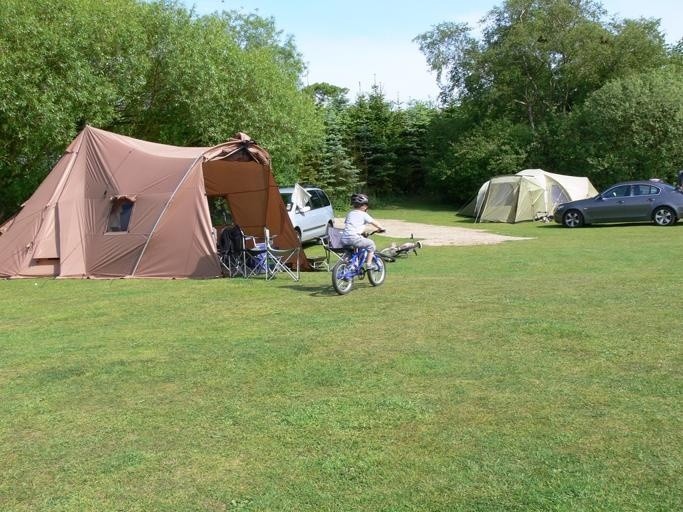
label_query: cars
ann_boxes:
[552,180,683,228]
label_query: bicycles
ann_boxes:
[331,229,387,295]
[379,234,422,258]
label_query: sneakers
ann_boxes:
[366,262,375,269]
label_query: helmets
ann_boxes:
[350,194,368,206]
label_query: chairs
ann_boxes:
[318,225,345,271]
[239,226,302,281]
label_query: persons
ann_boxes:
[340,194,382,270]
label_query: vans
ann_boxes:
[278,186,335,247]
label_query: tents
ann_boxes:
[0,124,315,279]
[456,169,600,223]
